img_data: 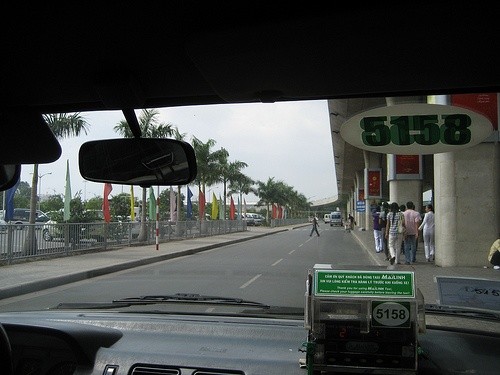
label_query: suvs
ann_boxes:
[42,209,106,242]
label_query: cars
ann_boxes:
[45,207,266,238]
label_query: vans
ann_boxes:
[8,208,50,230]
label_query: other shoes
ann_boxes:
[389,257,401,266]
[493,265,499,269]
[405,261,418,265]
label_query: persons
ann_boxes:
[372,201,434,264]
[309,215,320,237]
[488,240,500,269]
[348,214,356,228]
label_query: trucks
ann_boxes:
[323,214,331,224]
[328,211,344,227]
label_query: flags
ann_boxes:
[5,158,284,222]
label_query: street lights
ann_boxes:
[29,171,52,211]
